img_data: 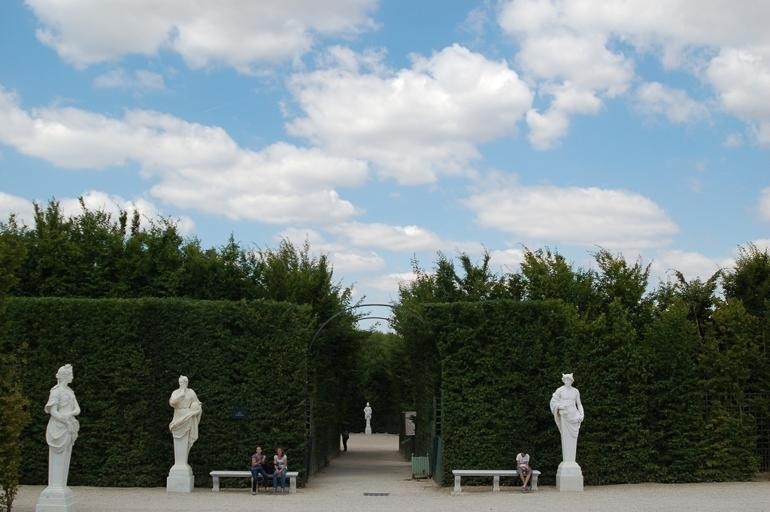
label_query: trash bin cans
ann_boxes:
[412,453,432,479]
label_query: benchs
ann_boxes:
[208,469,299,495]
[452,467,542,494]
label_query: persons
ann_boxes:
[341,422,349,450]
[363,401,372,428]
[249,446,268,494]
[43,363,80,489]
[515,449,532,491]
[549,372,584,461]
[271,449,287,492]
[169,374,203,466]
